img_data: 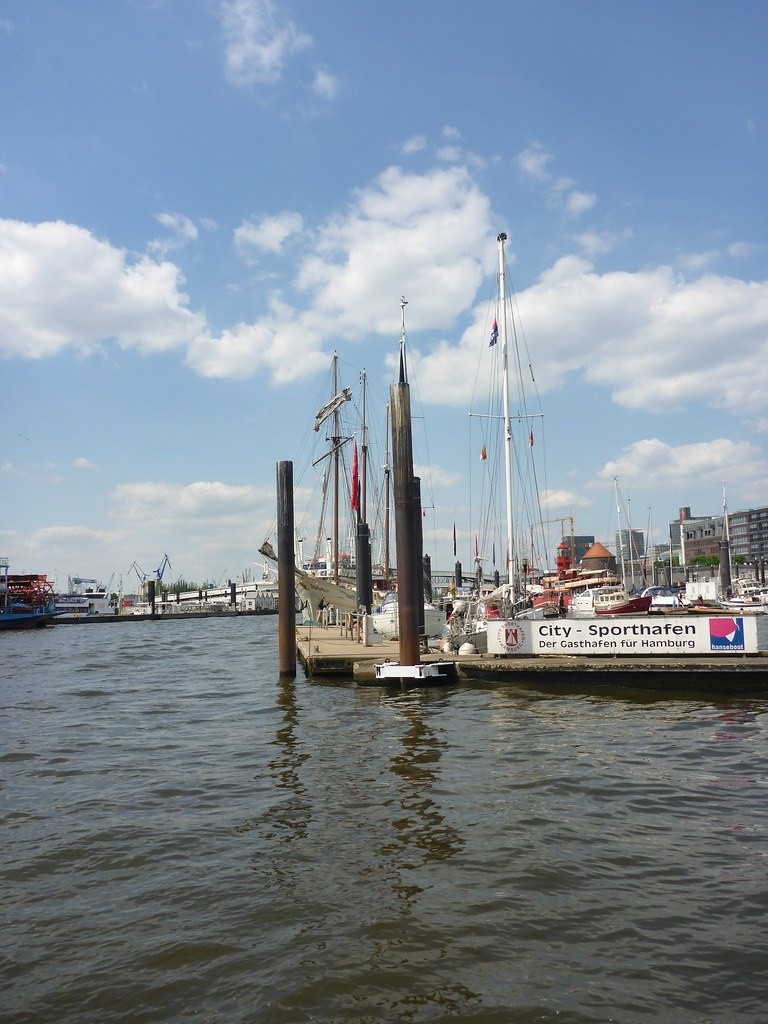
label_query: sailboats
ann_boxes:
[256,231,767,656]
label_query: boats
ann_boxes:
[0,586,67,629]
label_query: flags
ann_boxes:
[493,543,495,566]
[454,519,456,556]
[475,530,478,557]
[480,446,486,460]
[506,550,508,570]
[351,440,359,510]
[488,320,498,347]
[529,430,533,448]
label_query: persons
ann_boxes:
[557,597,564,619]
[526,597,533,609]
